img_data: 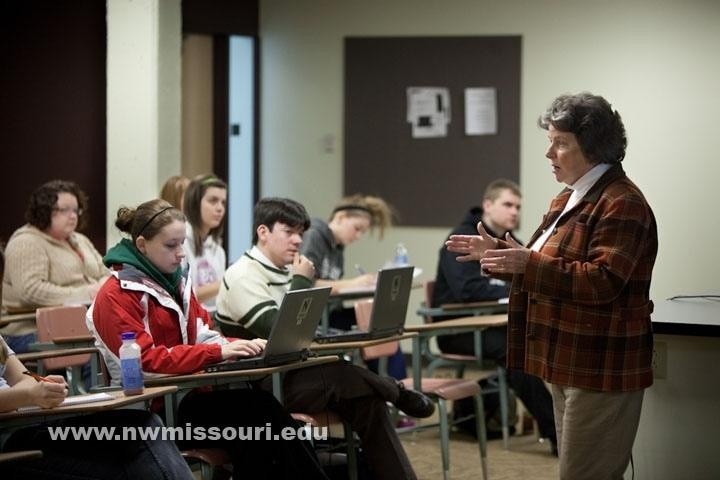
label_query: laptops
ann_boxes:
[203,287,332,373]
[313,266,414,344]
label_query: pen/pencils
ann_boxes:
[355,265,365,275]
[22,371,72,390]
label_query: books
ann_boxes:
[14,392,114,413]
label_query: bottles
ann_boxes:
[196,258,220,308]
[393,240,409,267]
[120,329,145,397]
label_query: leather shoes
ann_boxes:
[394,386,434,418]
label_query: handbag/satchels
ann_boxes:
[452,376,519,441]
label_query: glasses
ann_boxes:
[55,207,83,217]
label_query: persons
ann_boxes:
[211,192,435,480]
[428,180,560,453]
[160,170,201,214]
[86,200,331,478]
[444,90,661,478]
[0,248,196,480]
[180,176,234,344]
[1,179,115,393]
[301,192,414,436]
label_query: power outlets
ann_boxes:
[651,341,667,379]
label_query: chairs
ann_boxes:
[1,270,542,479]
